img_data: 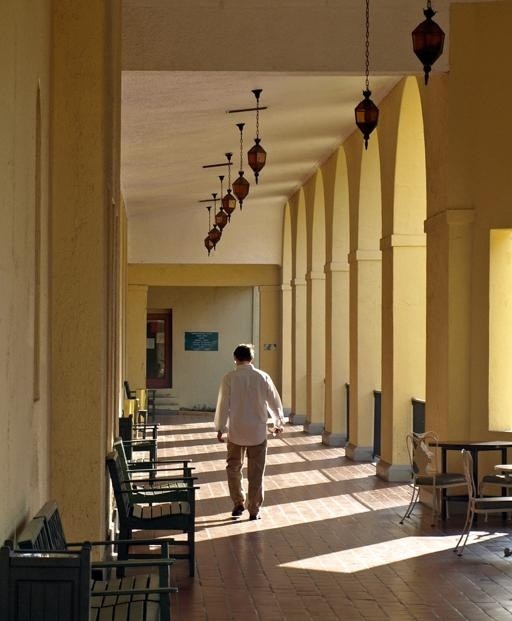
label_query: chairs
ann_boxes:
[121,379,161,435]
[400,430,511,558]
[1,497,180,621]
[106,435,200,586]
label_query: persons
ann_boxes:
[214,344,285,519]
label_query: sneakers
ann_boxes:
[232,502,244,516]
[249,512,261,520]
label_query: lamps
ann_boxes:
[353,1,381,154]
[202,85,268,259]
[409,1,446,88]
